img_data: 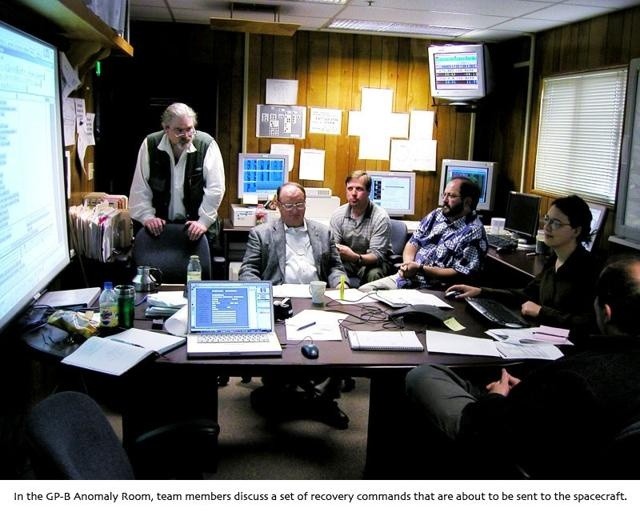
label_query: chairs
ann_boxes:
[461,398,640,480]
[24,389,140,480]
[130,222,213,284]
[389,219,409,258]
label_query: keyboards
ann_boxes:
[464,296,537,329]
[486,234,518,249]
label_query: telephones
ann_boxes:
[389,305,454,330]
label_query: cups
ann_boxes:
[115,285,136,329]
[307,281,327,304]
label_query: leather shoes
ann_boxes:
[261,392,349,426]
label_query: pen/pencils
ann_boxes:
[111,339,145,349]
[532,332,567,338]
[297,322,316,331]
[340,275,344,300]
[135,296,147,307]
[497,348,507,358]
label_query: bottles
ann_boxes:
[99,282,120,328]
[255,203,268,226]
[535,228,552,256]
[488,217,507,236]
[185,256,202,296]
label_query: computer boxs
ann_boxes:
[230,204,281,227]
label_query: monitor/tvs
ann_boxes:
[366,170,416,217]
[427,44,497,106]
[504,191,542,239]
[186,280,283,357]
[237,153,289,202]
[581,202,610,253]
[438,159,498,211]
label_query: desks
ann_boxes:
[406,228,547,280]
[23,281,568,480]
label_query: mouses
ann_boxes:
[301,344,320,360]
[496,246,508,253]
[444,290,461,300]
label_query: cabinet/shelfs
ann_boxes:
[76,247,132,287]
[220,217,254,281]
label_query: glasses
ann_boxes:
[169,123,197,138]
[440,192,467,199]
[278,199,306,212]
[544,213,570,229]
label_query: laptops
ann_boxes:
[404,220,421,233]
